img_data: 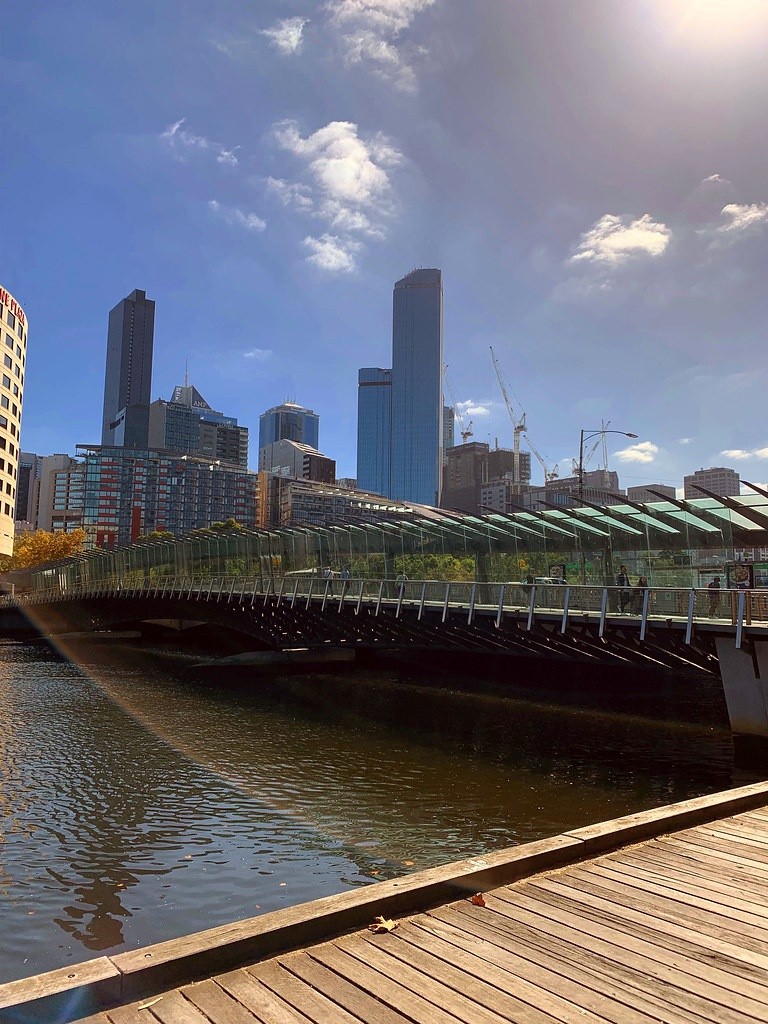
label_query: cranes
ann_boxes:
[490,344,560,485]
[442,365,474,444]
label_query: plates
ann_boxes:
[730,569,750,584]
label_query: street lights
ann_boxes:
[579,429,638,509]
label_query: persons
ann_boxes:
[341,565,350,599]
[395,571,408,603]
[323,567,333,599]
[522,575,537,610]
[707,577,720,619]
[2,593,29,604]
[117,578,123,590]
[632,577,650,616]
[60,587,66,595]
[615,566,631,614]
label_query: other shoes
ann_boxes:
[617,605,622,613]
[708,615,718,619]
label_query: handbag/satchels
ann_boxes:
[323,577,327,584]
